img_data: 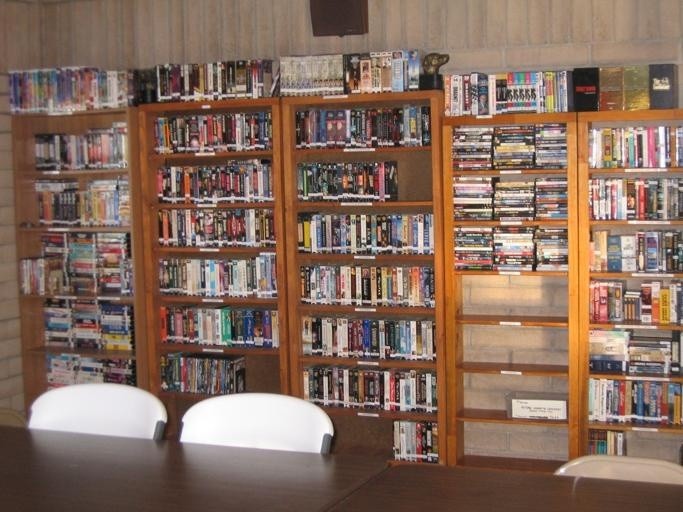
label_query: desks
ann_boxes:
[0,425,679,510]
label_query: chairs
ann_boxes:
[178,391,335,455]
[28,382,168,443]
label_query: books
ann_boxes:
[445,71,569,272]
[588,125,683,456]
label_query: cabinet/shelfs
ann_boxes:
[442,111,578,462]
[133,96,290,426]
[575,111,683,467]
[280,92,447,465]
[11,107,136,420]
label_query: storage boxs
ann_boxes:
[505,390,569,421]
